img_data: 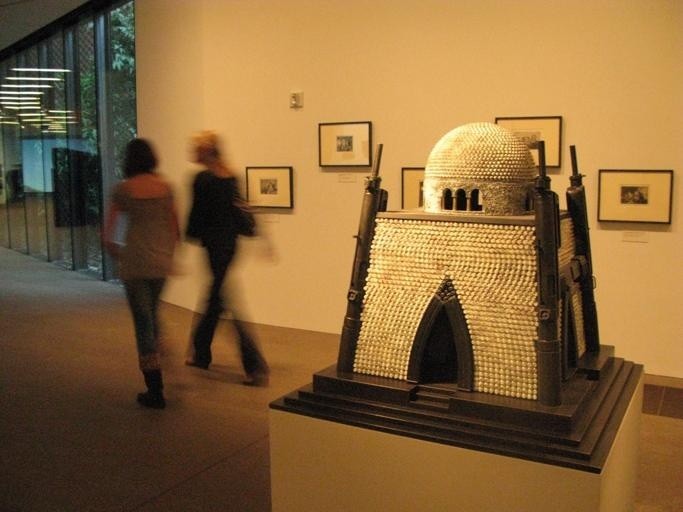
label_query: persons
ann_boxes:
[101,138,183,410]
[180,127,271,386]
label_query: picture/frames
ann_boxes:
[598,168,674,224]
[245,165,292,209]
[400,167,424,211]
[319,122,370,167]
[494,116,562,169]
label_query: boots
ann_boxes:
[137,369,167,409]
